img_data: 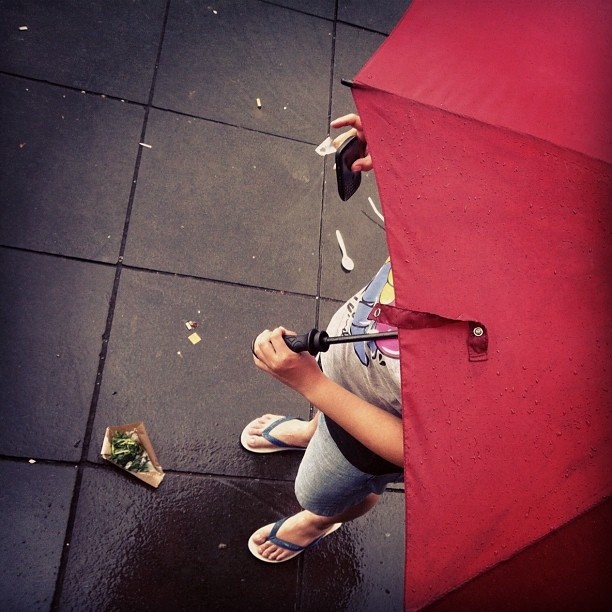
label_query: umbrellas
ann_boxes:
[250,0,612,612]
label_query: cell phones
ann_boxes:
[335,135,362,202]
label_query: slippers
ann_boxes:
[241,416,310,454]
[248,512,342,564]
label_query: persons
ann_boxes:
[239,113,404,566]
